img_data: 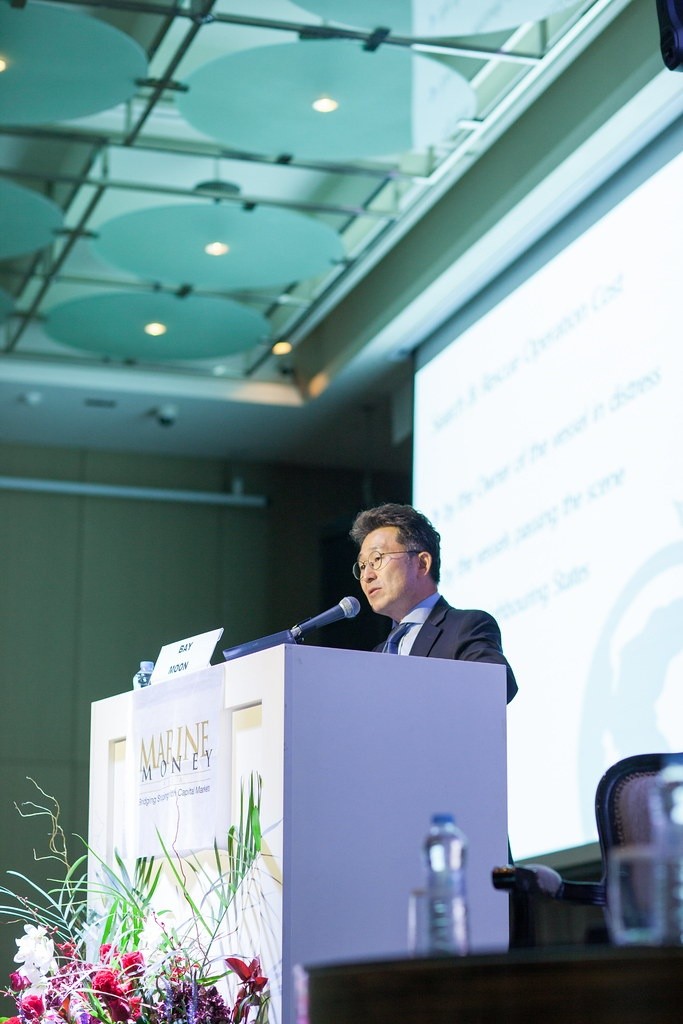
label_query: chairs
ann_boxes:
[493,751,683,947]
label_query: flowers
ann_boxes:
[0,771,279,1024]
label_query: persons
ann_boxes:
[347,502,542,948]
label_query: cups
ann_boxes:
[607,842,655,945]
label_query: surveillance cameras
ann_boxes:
[154,403,177,428]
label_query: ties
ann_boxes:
[382,623,410,654]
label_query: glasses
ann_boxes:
[353,550,420,580]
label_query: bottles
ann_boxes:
[653,753,683,946]
[133,661,154,690]
[424,812,468,957]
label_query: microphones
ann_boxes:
[290,595,362,638]
